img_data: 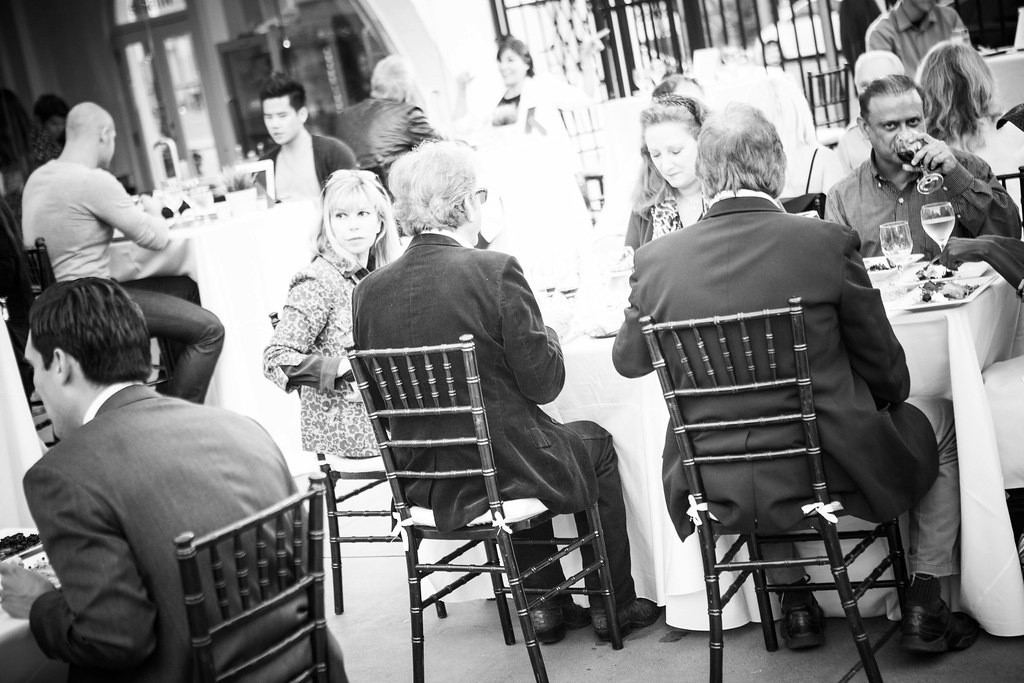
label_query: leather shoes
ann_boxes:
[589,596,659,644]
[531,600,591,644]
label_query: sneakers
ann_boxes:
[780,592,827,651]
[900,597,980,654]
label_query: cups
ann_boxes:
[189,185,214,215]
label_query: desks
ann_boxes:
[105,191,329,420]
[482,124,591,247]
[536,255,1024,638]
[0,526,77,683]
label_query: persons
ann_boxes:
[2,275,348,683]
[263,169,395,460]
[613,107,977,662]
[621,1,1024,314]
[350,140,657,646]
[258,36,570,202]
[9,93,225,407]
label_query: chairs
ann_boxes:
[0,63,1024,683]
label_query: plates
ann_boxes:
[0,528,43,564]
[892,261,989,290]
[862,254,924,282]
[900,275,999,313]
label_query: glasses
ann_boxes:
[470,187,488,204]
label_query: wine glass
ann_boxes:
[920,201,956,253]
[894,129,944,195]
[879,220,914,295]
[158,178,182,224]
